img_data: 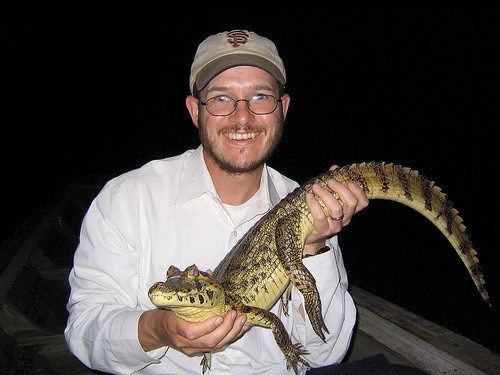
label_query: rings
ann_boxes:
[329,214,344,222]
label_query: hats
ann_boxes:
[189,30,286,96]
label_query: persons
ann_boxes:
[63,29,372,375]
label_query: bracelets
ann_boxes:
[301,245,330,260]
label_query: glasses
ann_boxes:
[199,95,282,116]
